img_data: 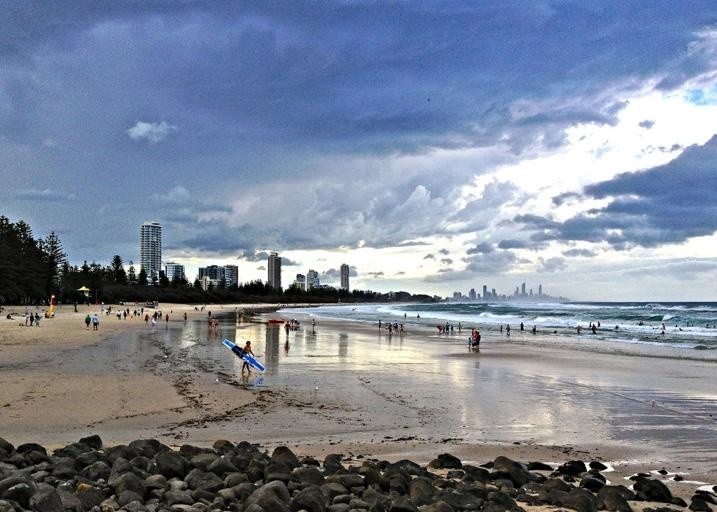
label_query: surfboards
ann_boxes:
[223,339,265,372]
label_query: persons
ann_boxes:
[312,319,316,334]
[378,313,421,336]
[7,303,55,327]
[284,335,291,355]
[239,340,254,372]
[239,312,242,318]
[284,320,291,335]
[436,318,694,351]
[84,299,221,330]
